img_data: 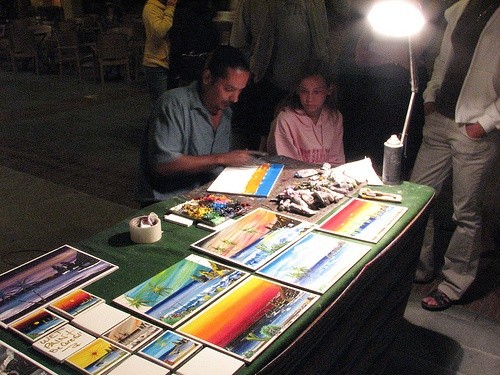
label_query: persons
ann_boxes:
[136,46,256,209]
[230,0,353,152]
[408,0,500,310]
[268,61,346,169]
[141,0,177,108]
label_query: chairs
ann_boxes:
[0,14,150,84]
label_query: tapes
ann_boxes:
[128,215,163,244]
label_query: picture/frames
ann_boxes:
[174,275,321,363]
[206,162,284,198]
[112,254,251,328]
[314,197,409,245]
[190,206,318,271]
[64,337,131,375]
[101,316,164,354]
[255,232,372,296]
[358,188,403,203]
[0,244,119,329]
[137,329,204,371]
[49,289,106,321]
[107,354,170,375]
[30,324,96,364]
[176,347,245,375]
[9,308,69,343]
[70,304,131,338]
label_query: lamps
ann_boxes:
[366,0,428,186]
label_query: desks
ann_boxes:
[0,153,437,375]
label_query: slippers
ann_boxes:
[421,288,454,310]
